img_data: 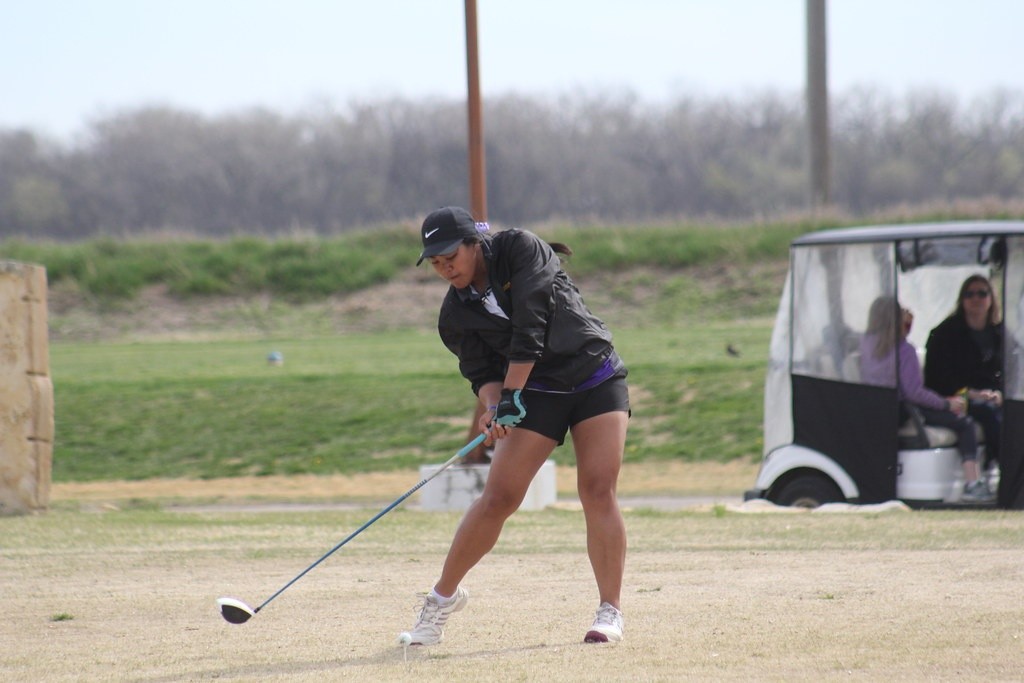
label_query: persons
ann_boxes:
[924,274,1003,493]
[410,206,633,645]
[861,296,996,501]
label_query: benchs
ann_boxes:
[844,347,986,450]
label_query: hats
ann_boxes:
[416,206,476,266]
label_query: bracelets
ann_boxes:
[488,405,496,410]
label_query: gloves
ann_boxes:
[486,388,528,430]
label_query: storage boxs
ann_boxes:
[417,457,557,512]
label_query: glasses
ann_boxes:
[965,290,987,298]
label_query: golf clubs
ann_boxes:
[213,422,497,625]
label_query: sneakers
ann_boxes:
[960,478,998,501]
[584,602,624,643]
[408,585,469,646]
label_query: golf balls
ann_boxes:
[398,633,412,647]
